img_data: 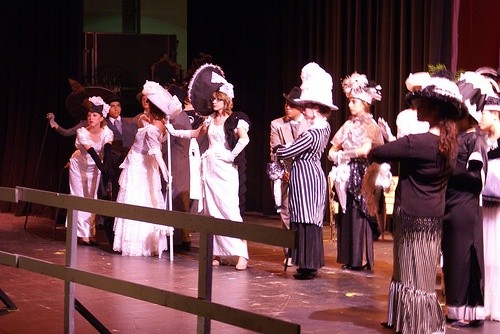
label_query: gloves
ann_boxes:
[164,124,191,140]
[215,138,249,162]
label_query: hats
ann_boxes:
[283,87,304,107]
[342,71,382,105]
[405,66,500,125]
[143,80,182,118]
[293,62,339,110]
[189,63,234,116]
[82,97,109,118]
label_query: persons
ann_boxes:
[165,64,250,271]
[270,61,500,334]
[46,82,202,259]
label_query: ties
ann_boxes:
[115,120,122,134]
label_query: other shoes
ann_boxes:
[343,260,369,270]
[293,268,316,279]
[445,314,458,322]
[283,258,293,266]
[235,258,247,270]
[213,256,222,266]
[381,320,392,328]
[81,236,99,245]
[470,320,484,327]
